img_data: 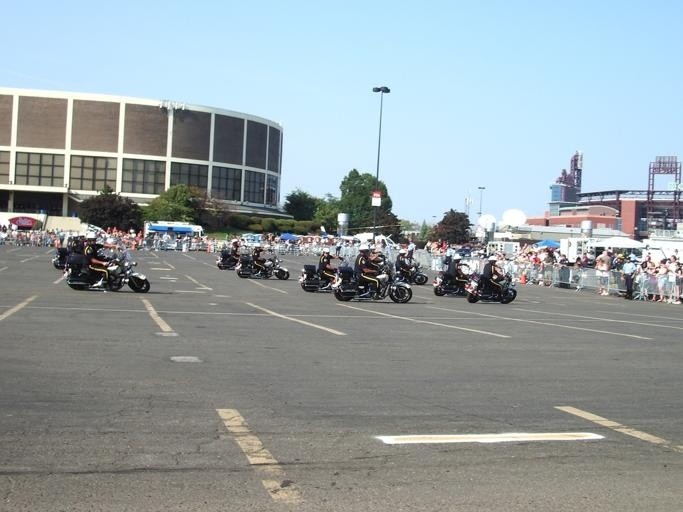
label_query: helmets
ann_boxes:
[399,249,406,254]
[488,256,497,261]
[323,247,329,253]
[86,232,97,238]
[359,244,369,251]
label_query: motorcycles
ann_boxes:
[215,240,259,270]
[236,242,291,283]
[47,230,149,294]
[385,255,430,285]
[434,260,477,297]
[334,255,414,304]
[298,253,353,295]
[464,263,520,305]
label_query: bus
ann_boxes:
[142,220,204,244]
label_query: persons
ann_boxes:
[2,226,143,250]
[267,232,417,263]
[317,248,345,288]
[572,246,681,301]
[145,232,216,253]
[224,232,266,273]
[483,256,506,298]
[353,244,385,299]
[423,238,570,289]
[64,233,121,283]
[395,249,414,282]
[447,253,469,295]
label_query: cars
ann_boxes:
[455,248,469,257]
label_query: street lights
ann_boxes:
[478,185,488,212]
[370,84,391,241]
